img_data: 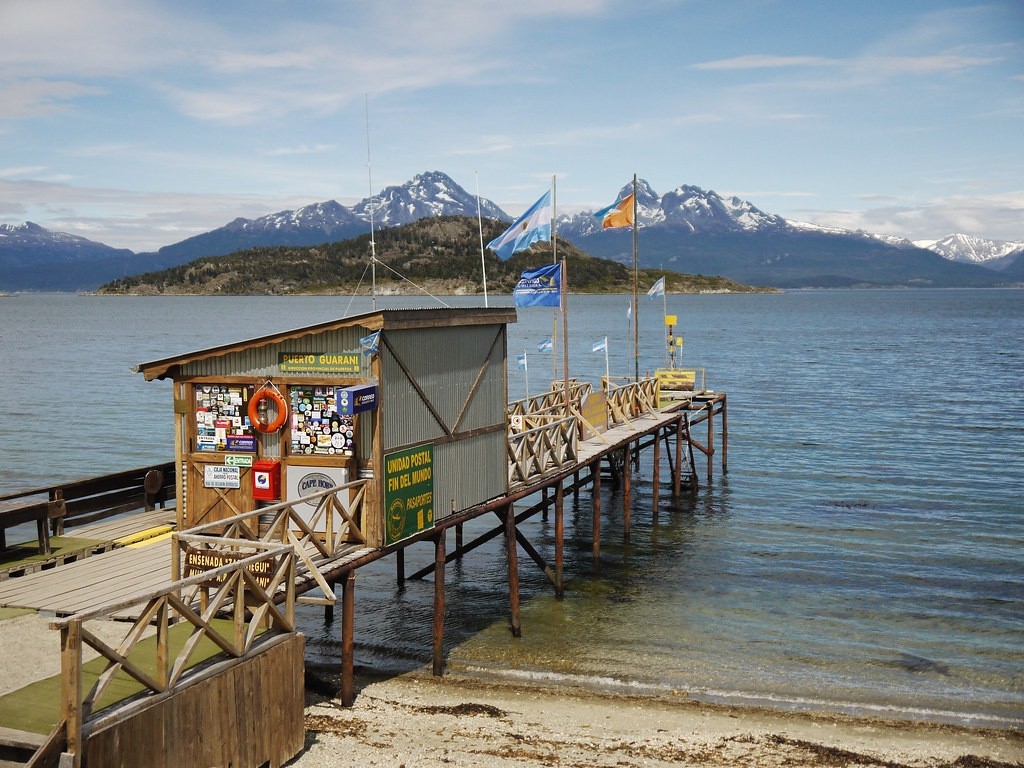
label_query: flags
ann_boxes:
[485,187,554,262]
[537,337,554,354]
[646,276,665,301]
[516,353,526,370]
[512,259,564,310]
[626,300,633,321]
[592,340,607,353]
[592,193,636,230]
[358,331,382,358]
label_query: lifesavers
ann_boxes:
[248,388,289,436]
